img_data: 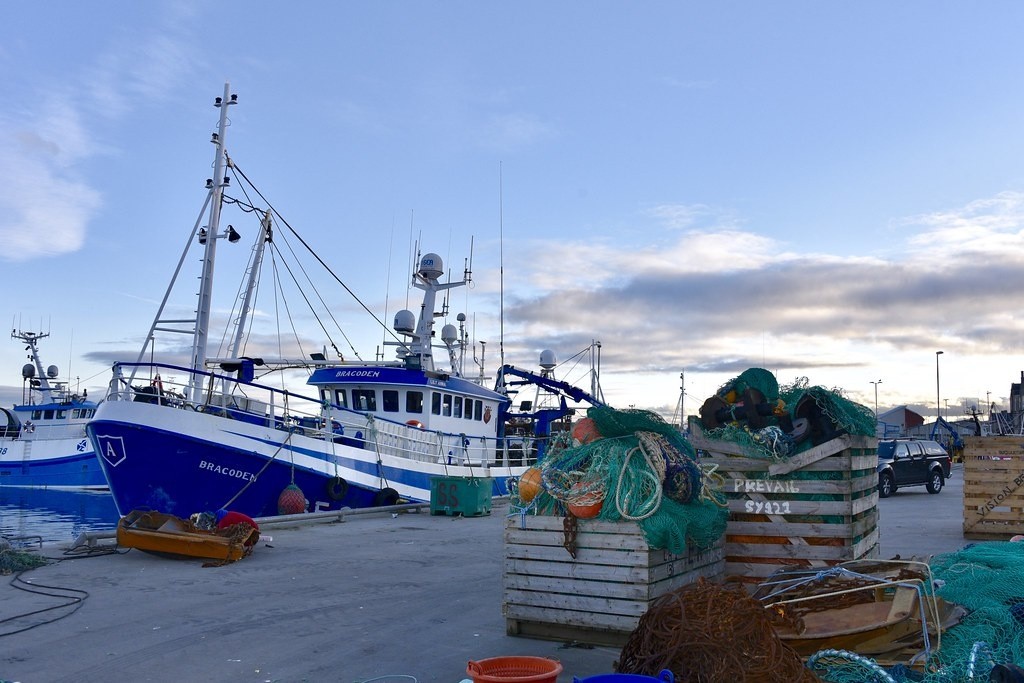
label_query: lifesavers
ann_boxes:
[24,422,35,433]
[406,420,425,433]
[374,488,400,507]
[326,477,348,502]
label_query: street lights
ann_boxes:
[934,350,943,443]
[870,381,883,422]
[944,398,949,423]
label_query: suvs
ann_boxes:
[877,436,952,497]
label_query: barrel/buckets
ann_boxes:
[572,669,674,683]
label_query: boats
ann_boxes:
[79,69,590,524]
[0,307,112,493]
